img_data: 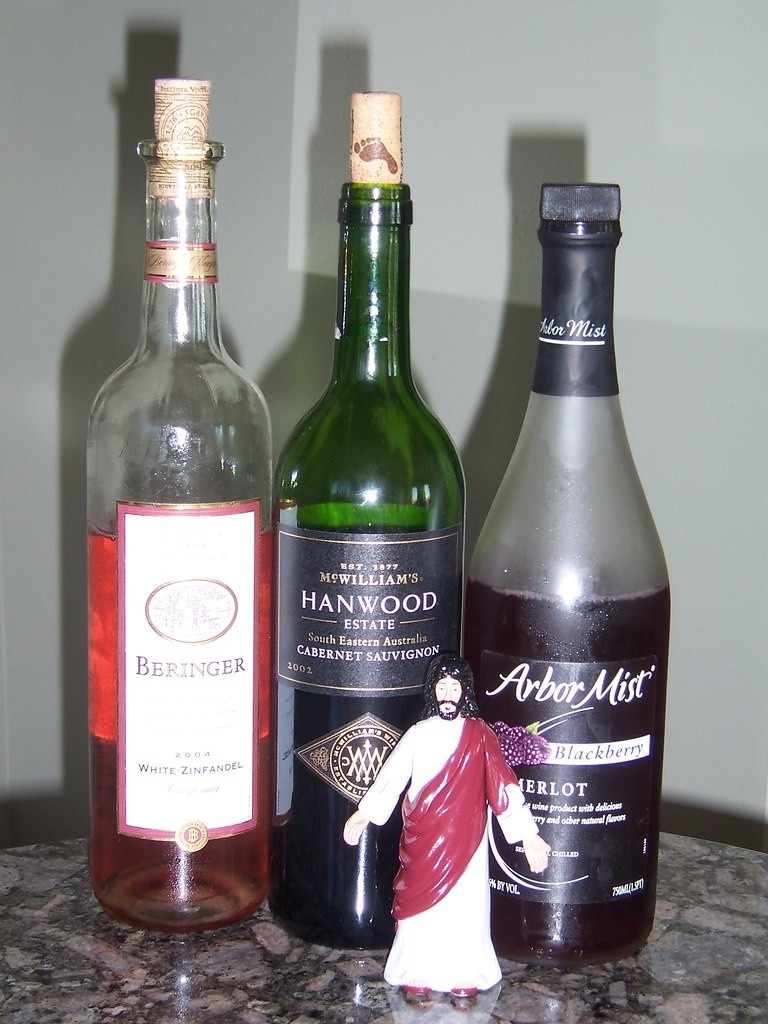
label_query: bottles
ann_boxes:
[86,76,277,930]
[466,183,671,969]
[273,91,470,955]
